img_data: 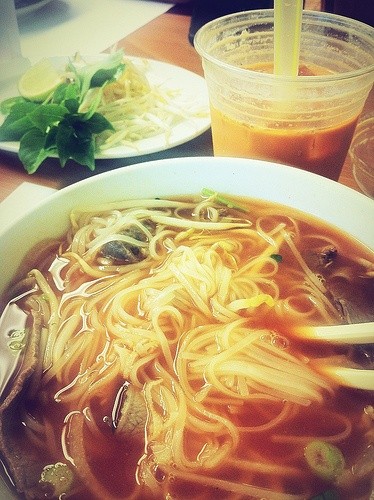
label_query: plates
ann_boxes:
[0,53,212,159]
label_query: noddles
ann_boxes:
[0,192,374,500]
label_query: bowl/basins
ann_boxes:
[0,157,373,500]
[348,108,374,201]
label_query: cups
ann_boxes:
[193,8,374,181]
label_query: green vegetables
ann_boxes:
[0,46,126,175]
